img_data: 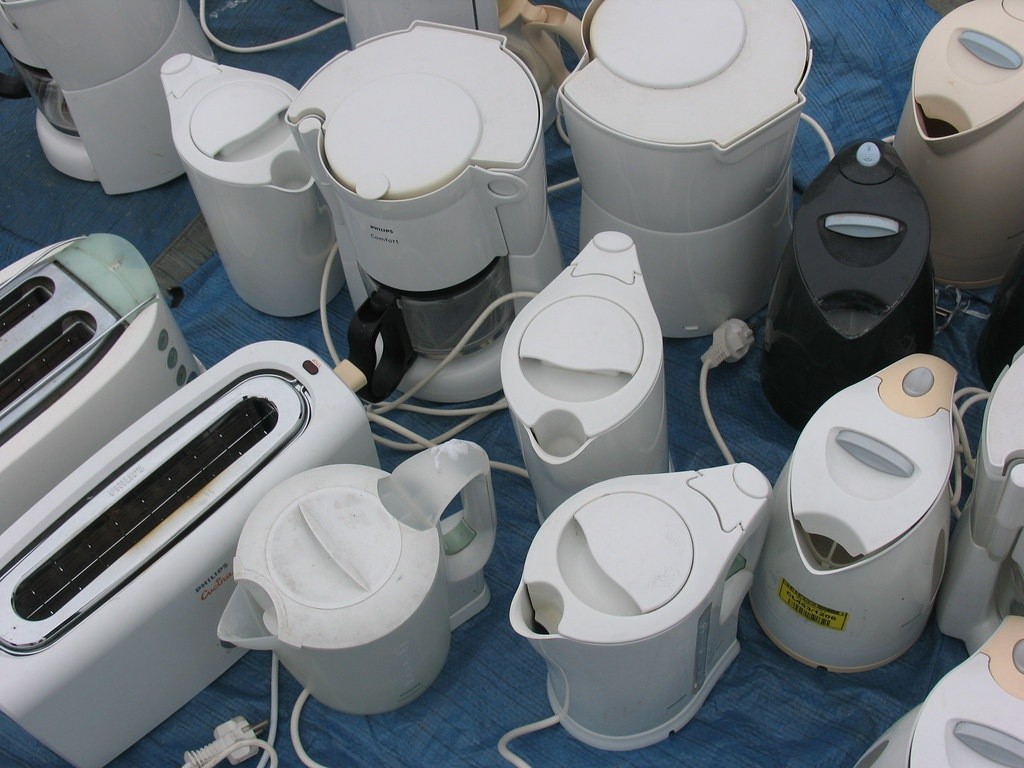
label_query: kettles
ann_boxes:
[1,0,1024,768]
